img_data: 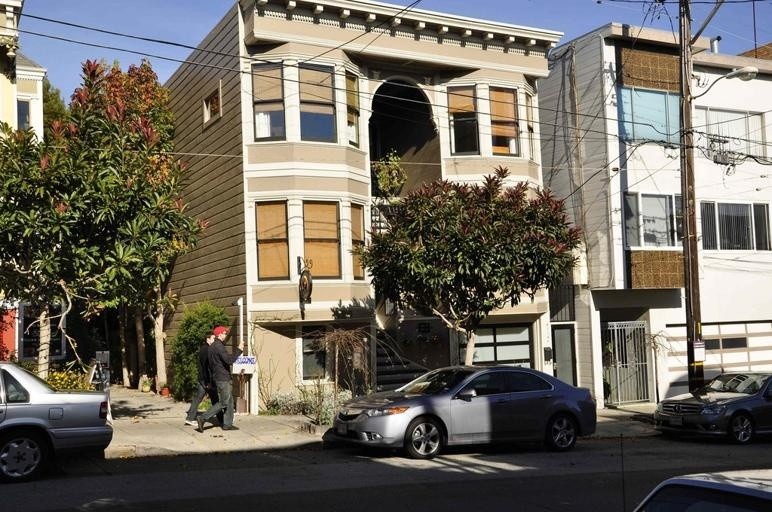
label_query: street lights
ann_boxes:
[681,64,759,391]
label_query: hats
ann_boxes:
[214,326,228,336]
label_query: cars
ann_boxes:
[652,371,772,443]
[633,469,771,512]
[322,364,598,460]
[0,361,114,481]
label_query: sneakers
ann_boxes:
[185,419,198,426]
[197,415,204,432]
[223,425,238,430]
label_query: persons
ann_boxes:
[186,332,224,427]
[197,324,246,433]
